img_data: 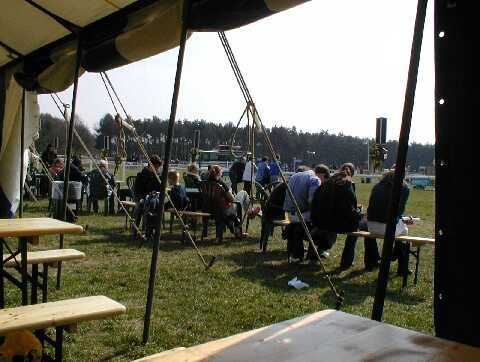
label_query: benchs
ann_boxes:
[119,200,210,237]
[0,295,126,362]
[3,248,86,304]
[274,220,435,284]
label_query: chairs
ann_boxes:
[85,171,114,215]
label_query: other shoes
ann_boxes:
[250,207,259,219]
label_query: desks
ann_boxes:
[135,306,480,362]
[0,217,83,309]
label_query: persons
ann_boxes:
[25,144,114,214]
[329,163,381,272]
[133,155,260,239]
[283,164,337,258]
[263,166,310,220]
[229,154,282,201]
[366,163,413,276]
